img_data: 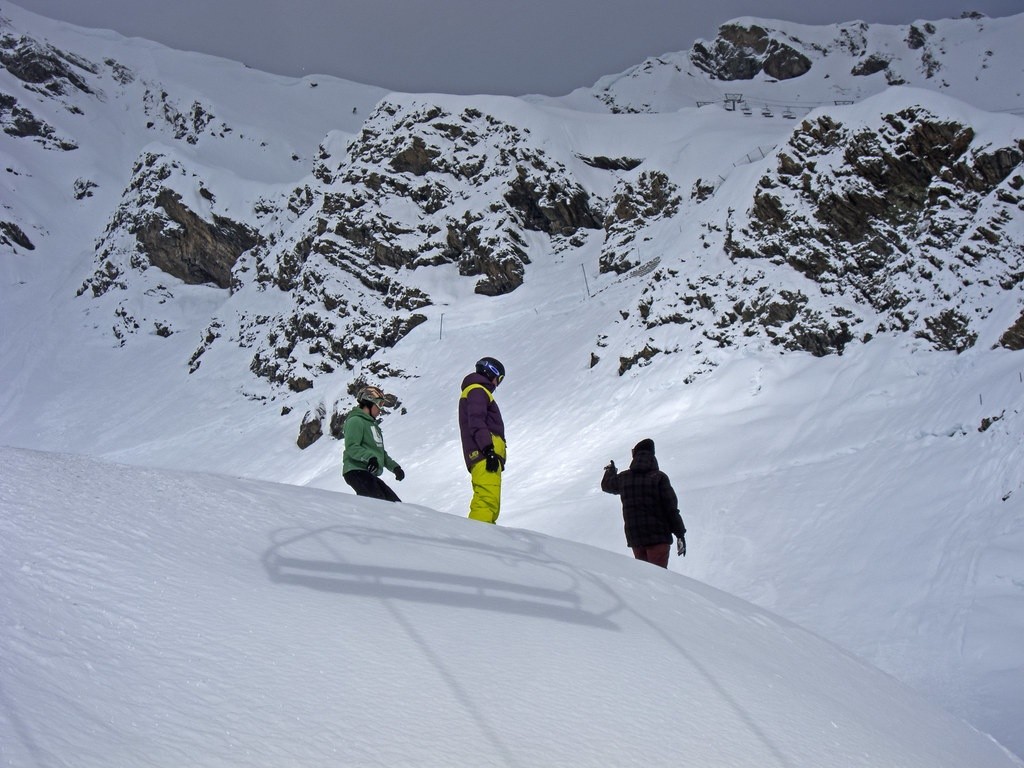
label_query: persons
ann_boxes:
[458,357,507,524]
[600,437,686,569]
[340,386,405,502]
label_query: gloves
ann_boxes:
[677,536,686,557]
[604,460,618,472]
[482,443,499,472]
[393,466,405,481]
[367,457,379,475]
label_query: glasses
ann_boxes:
[632,449,654,458]
[476,360,504,386]
[363,396,384,409]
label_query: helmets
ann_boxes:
[357,386,384,403]
[476,357,505,382]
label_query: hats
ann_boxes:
[633,439,655,458]
